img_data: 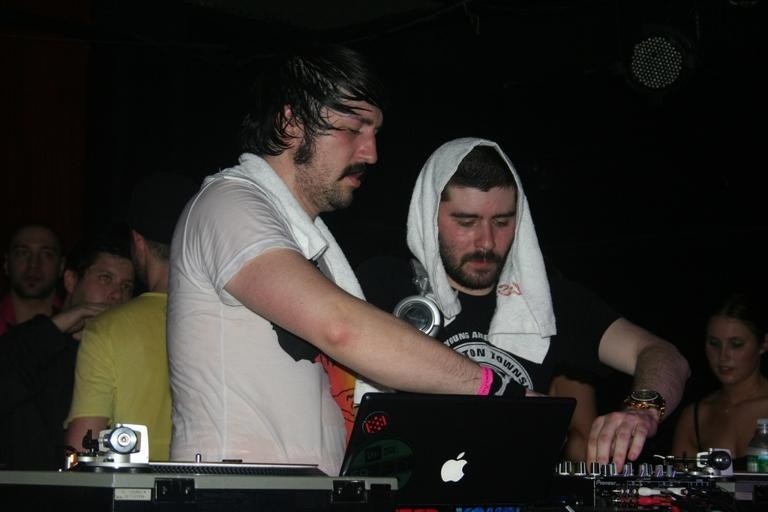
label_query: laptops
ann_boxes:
[336,391,577,502]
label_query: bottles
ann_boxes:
[747,418,768,473]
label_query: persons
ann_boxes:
[162,37,569,477]
[351,136,695,473]
